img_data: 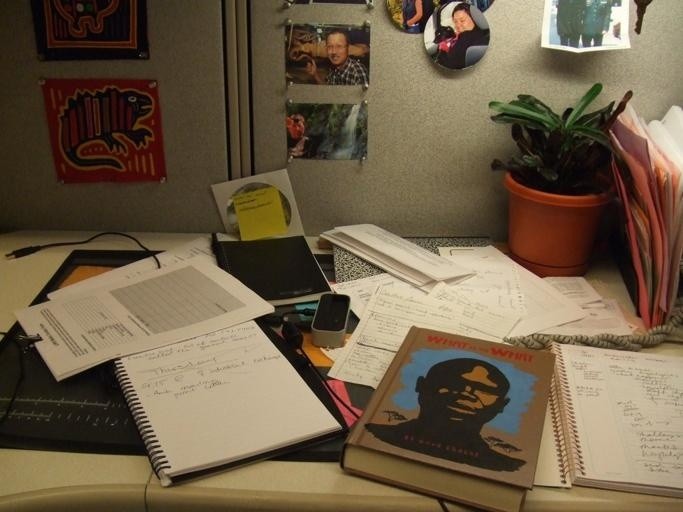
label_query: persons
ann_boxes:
[437,4,483,69]
[557,0,585,48]
[583,0,610,47]
[307,31,370,85]
[404,0,423,33]
[369,358,527,471]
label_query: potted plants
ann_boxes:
[486,83,634,279]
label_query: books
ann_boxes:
[339,325,556,511]
[610,103,683,331]
[218,234,333,305]
[534,342,683,498]
[114,320,351,488]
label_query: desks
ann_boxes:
[0,228,682,512]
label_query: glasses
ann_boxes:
[326,45,347,50]
